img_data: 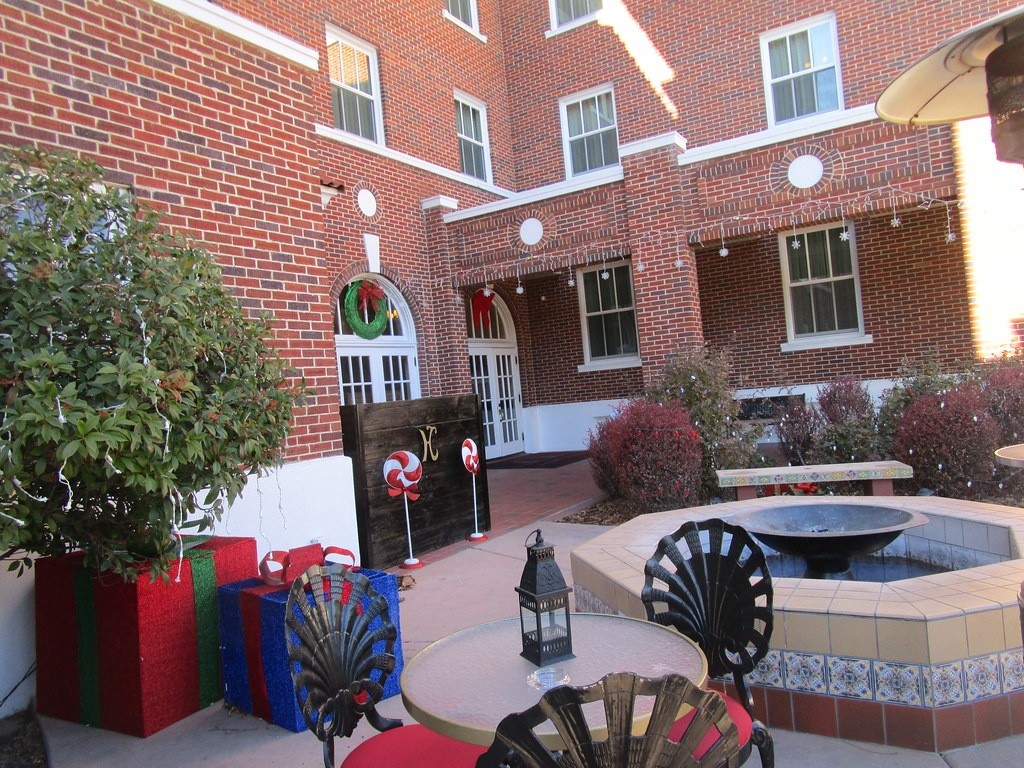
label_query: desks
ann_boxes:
[400,611,708,752]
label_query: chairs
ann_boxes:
[283,564,489,768]
[641,518,775,768]
[473,671,739,768]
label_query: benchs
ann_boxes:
[715,460,914,504]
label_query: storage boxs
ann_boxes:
[217,558,403,734]
[35,534,258,738]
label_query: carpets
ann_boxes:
[486,450,593,469]
